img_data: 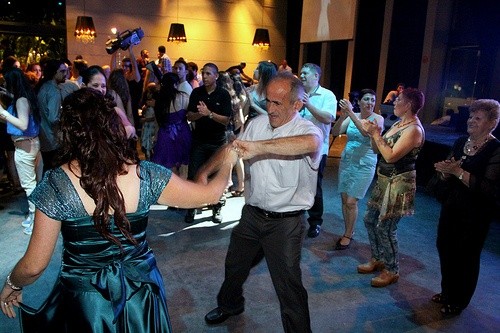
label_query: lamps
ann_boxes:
[252,0,271,47]
[166,0,188,43]
[74,0,98,44]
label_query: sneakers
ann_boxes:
[23,223,33,236]
[21,214,32,228]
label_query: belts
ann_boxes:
[15,136,38,142]
[249,205,306,218]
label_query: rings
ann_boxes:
[4,302,8,305]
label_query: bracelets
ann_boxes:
[458,170,466,181]
[6,273,22,290]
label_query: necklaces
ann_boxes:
[398,117,418,128]
[465,135,492,152]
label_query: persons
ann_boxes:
[194,73,323,333]
[384,83,405,106]
[217,60,291,197]
[0,56,136,235]
[298,63,337,238]
[0,87,242,333]
[109,30,199,210]
[358,88,424,286]
[332,89,384,249]
[433,99,500,313]
[185,63,233,222]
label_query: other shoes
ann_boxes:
[334,234,353,250]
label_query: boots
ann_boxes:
[212,204,222,223]
[184,209,195,223]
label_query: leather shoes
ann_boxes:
[432,293,446,303]
[371,269,399,287]
[436,304,465,317]
[307,225,321,237]
[205,306,245,325]
[358,258,385,273]
